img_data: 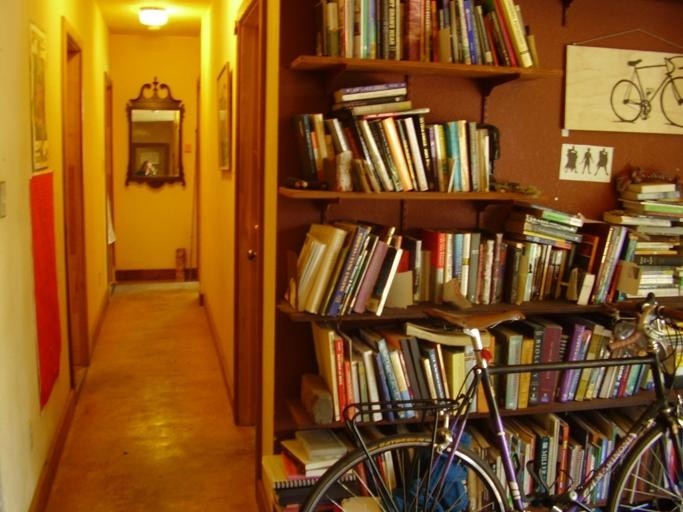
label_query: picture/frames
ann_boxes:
[27,20,51,173]
[215,62,232,171]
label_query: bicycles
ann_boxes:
[299,293,682,510]
[610,54,683,127]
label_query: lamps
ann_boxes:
[135,5,175,33]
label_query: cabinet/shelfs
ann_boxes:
[276,56,683,431]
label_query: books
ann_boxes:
[263,404,682,512]
[311,1,539,68]
[289,82,493,192]
[283,199,637,317]
[310,319,683,423]
[602,180,683,299]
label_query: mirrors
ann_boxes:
[125,78,185,189]
